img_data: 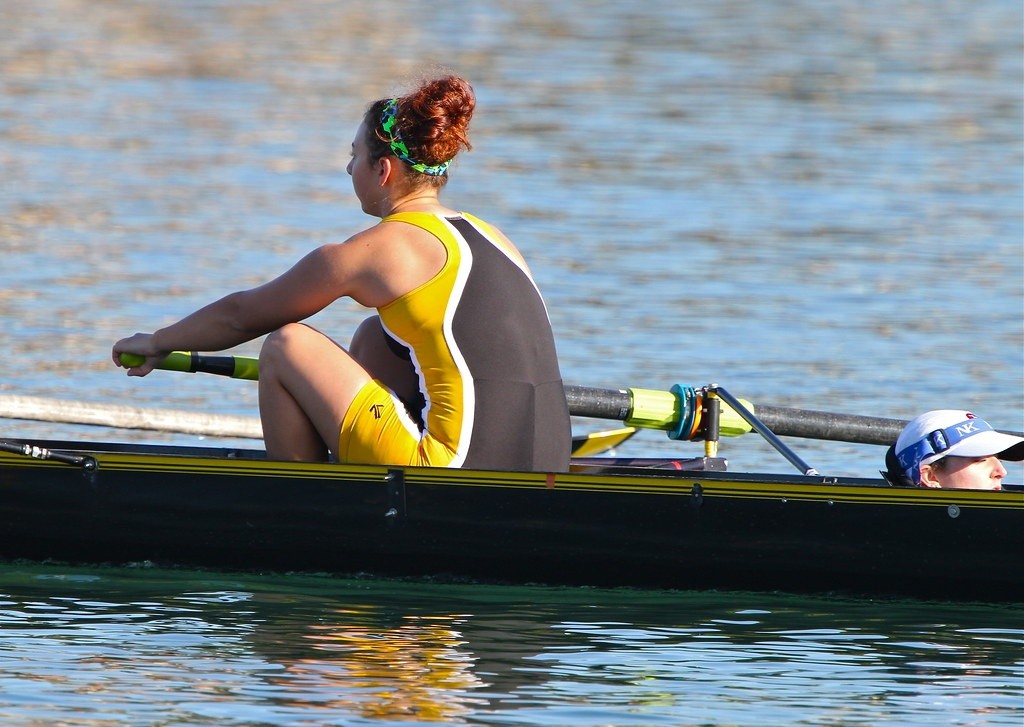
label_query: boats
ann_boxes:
[1,414,1024,615]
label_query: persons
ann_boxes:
[112,71,571,473]
[877,409,1024,490]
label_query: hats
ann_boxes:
[895,409,1024,488]
